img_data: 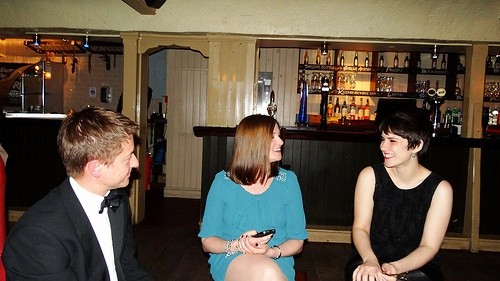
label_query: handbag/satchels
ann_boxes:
[397,269,427,281]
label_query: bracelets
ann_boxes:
[271,245,282,260]
[225,239,235,256]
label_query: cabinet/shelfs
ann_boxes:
[297,49,500,129]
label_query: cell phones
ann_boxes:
[253,228,276,238]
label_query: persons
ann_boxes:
[344,110,453,281]
[197,114,309,281]
[3,105,153,281]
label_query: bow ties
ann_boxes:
[100,191,123,214]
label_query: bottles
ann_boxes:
[481,55,500,131]
[297,48,464,127]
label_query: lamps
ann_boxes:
[84,37,91,47]
[33,35,41,46]
[321,44,328,54]
[432,47,439,58]
